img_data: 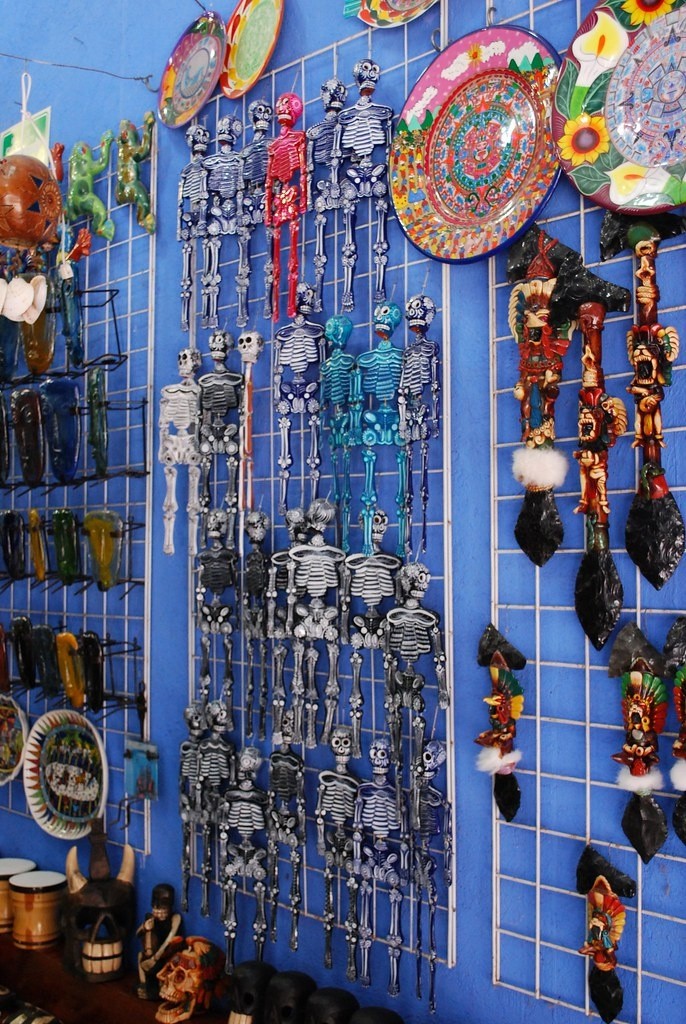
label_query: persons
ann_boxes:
[134,882,183,1002]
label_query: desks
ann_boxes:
[0,931,235,1024]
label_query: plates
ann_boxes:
[219,0,284,100]
[388,24,562,266]
[357,0,438,28]
[157,11,227,130]
[552,0,686,215]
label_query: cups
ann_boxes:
[0,858,36,934]
[9,871,67,950]
